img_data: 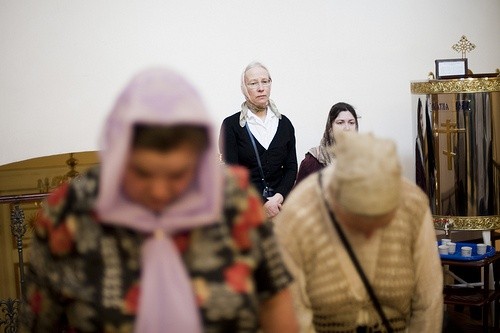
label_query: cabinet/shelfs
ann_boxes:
[440,251,500,333]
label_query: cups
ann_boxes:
[477,244,487,255]
[441,239,451,245]
[446,243,456,254]
[438,245,449,255]
[461,247,472,257]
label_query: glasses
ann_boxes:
[249,79,270,88]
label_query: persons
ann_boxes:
[217,62,298,216]
[17,66,300,333]
[295,101,359,194]
[272,131,444,333]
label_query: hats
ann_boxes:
[329,132,405,213]
